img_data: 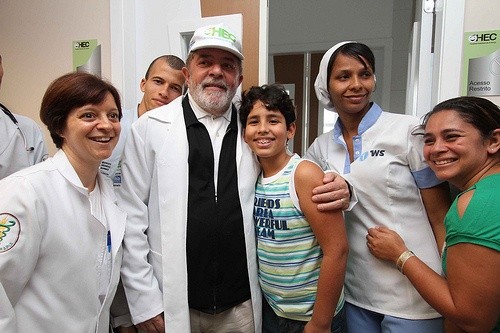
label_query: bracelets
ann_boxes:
[396,250,416,272]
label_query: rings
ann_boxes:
[340,199,346,207]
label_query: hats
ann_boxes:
[314,41,376,112]
[187,23,246,61]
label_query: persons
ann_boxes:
[367,95,500,333]
[0,52,50,178]
[121,55,186,128]
[304,40,450,333]
[121,23,358,333]
[0,71,128,333]
[238,84,350,333]
